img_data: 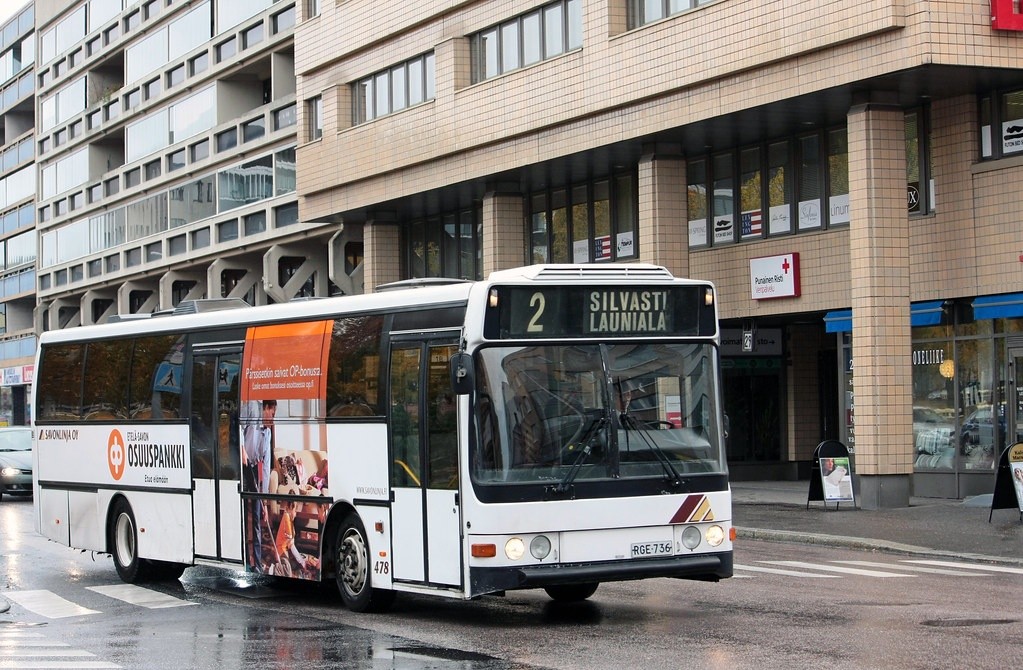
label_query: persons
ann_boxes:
[267,487,307,578]
[307,493,330,580]
[326,360,458,489]
[944,363,967,417]
[577,378,648,464]
[239,398,278,572]
[821,458,836,477]
[307,460,327,492]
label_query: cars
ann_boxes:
[0,426,33,500]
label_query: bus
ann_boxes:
[27,263,735,604]
